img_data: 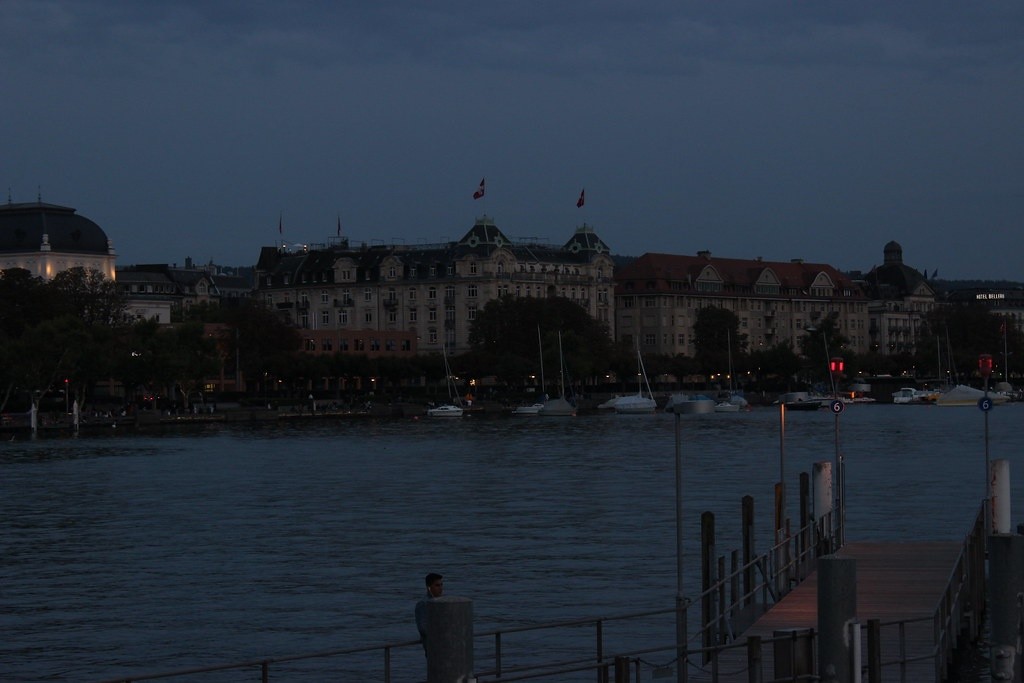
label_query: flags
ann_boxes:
[576,190,584,207]
[337,220,341,234]
[473,179,484,199]
[279,216,283,234]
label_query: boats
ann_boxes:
[935,382,1012,405]
[427,404,463,418]
[850,396,875,405]
[663,390,717,412]
[785,400,823,411]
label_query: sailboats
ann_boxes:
[713,328,752,413]
[821,328,854,405]
[892,332,960,406]
[439,344,486,416]
[597,335,658,415]
[511,322,579,416]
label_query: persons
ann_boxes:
[0,400,216,427]
[415,572,443,659]
[295,391,545,417]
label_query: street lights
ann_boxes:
[978,352,994,536]
[830,356,843,547]
